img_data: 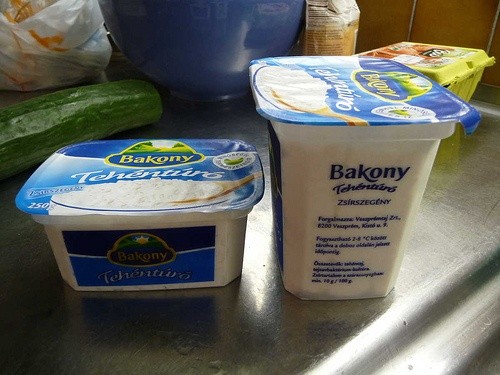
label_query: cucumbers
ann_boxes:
[0,78,163,181]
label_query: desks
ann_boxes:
[0,93,499,375]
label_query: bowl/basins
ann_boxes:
[97,0,306,101]
